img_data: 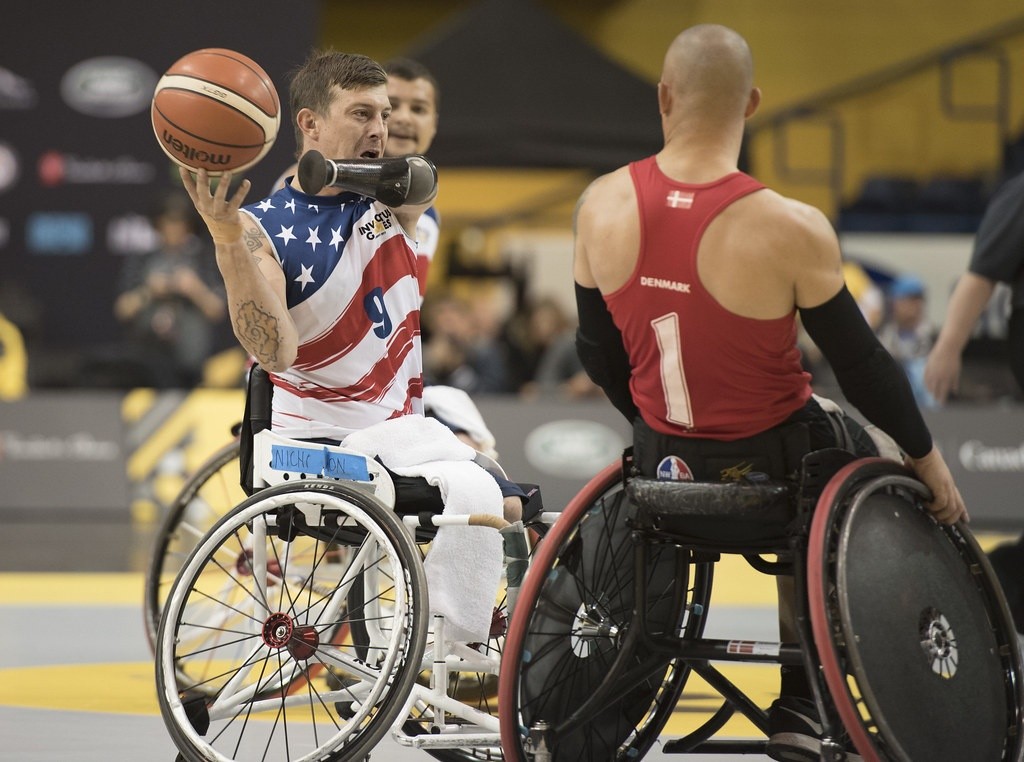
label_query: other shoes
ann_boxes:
[446,668,500,701]
[764,697,890,762]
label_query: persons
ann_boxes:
[180,53,522,527]
[0,177,1024,406]
[575,24,970,762]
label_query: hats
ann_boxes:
[893,276,925,298]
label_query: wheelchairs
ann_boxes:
[491,444,1024,762]
[154,356,562,762]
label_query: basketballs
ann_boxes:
[150,46,282,179]
